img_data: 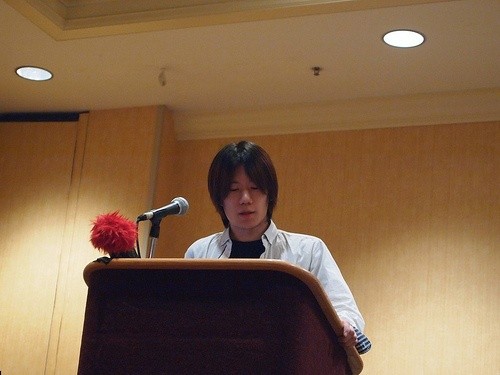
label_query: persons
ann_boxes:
[184,141,372,355]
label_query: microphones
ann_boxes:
[137,197,189,222]
